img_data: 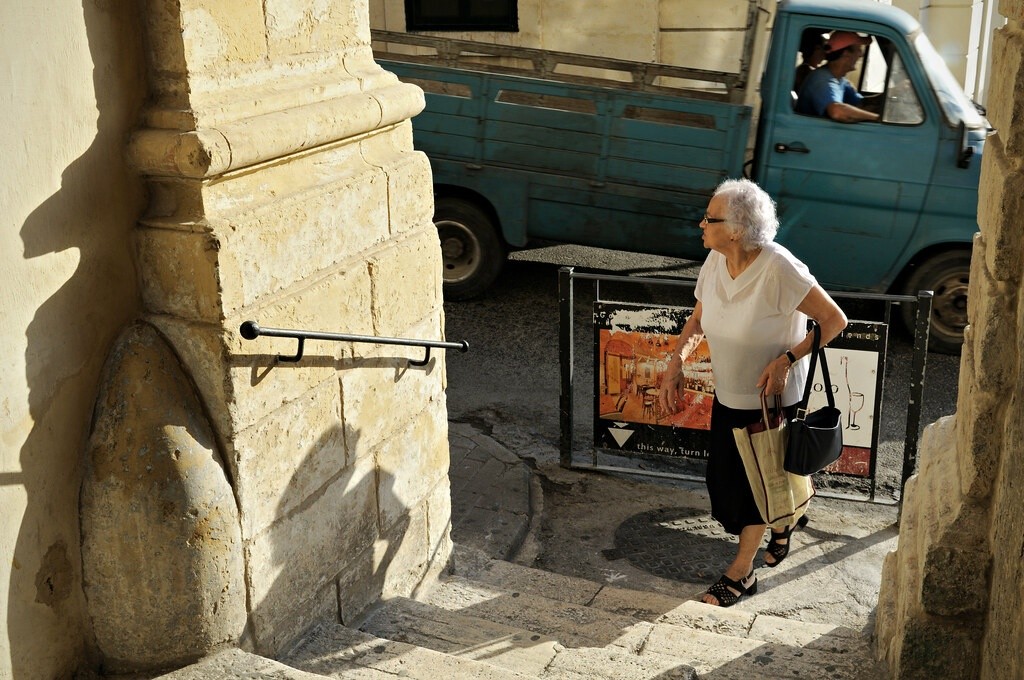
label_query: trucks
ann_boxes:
[368,0,984,353]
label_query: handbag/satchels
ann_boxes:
[785,323,844,475]
[732,383,815,528]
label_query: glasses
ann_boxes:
[703,214,729,225]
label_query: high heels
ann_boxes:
[701,569,757,606]
[766,514,808,567]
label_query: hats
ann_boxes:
[824,30,872,53]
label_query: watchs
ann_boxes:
[786,349,798,368]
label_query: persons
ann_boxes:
[795,29,910,122]
[659,180,847,608]
